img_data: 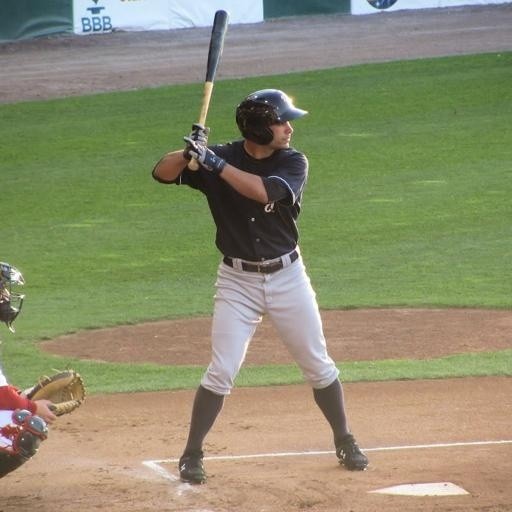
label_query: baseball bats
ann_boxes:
[187,10,228,172]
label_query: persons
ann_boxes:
[0,261,85,479]
[152,88,369,484]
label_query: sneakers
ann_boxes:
[179,450,206,482]
[336,434,369,471]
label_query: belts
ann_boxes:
[224,252,299,275]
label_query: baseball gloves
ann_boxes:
[20,370,87,418]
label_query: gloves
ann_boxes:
[183,125,226,173]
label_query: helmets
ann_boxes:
[235,89,309,145]
[0,263,19,322]
